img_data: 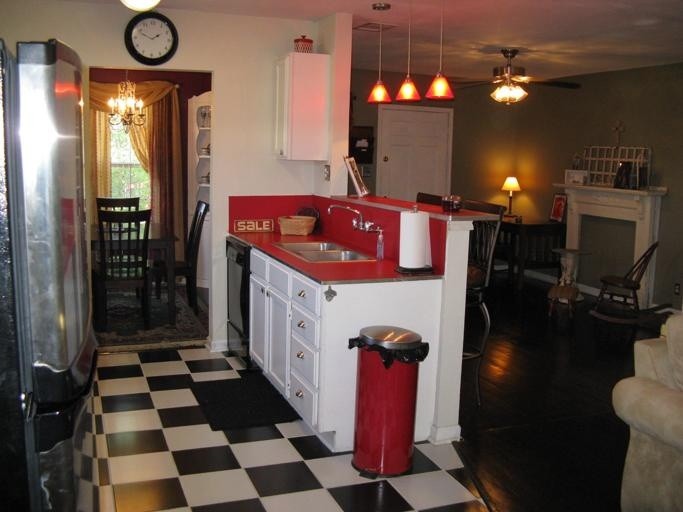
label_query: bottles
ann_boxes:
[293,34,312,54]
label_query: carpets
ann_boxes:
[90,268,212,348]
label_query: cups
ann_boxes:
[441,195,461,212]
[200,176,208,183]
[199,148,208,156]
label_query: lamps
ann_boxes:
[501,176,522,217]
[393,1,423,103]
[105,69,146,135]
[425,0,457,102]
[362,0,395,104]
[489,80,528,107]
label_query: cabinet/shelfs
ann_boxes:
[282,274,445,454]
[273,49,332,164]
[183,92,213,294]
[242,245,293,399]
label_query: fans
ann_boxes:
[446,41,585,97]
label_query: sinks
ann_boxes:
[272,241,341,251]
[296,250,376,263]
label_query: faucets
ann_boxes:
[327,204,364,228]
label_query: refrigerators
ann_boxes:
[0,36,105,511]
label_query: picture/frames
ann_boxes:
[548,192,567,223]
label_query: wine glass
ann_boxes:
[200,111,206,127]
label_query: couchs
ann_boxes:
[606,304,682,512]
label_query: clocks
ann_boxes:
[123,10,177,67]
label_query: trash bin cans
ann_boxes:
[348,326,429,480]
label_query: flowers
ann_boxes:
[565,152,587,171]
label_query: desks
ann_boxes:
[493,213,565,294]
[86,221,180,328]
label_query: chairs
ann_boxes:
[460,197,508,407]
[94,205,152,331]
[586,236,675,328]
[414,191,448,205]
[92,195,145,265]
[547,242,591,319]
[153,198,210,315]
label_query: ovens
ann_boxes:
[222,235,250,368]
